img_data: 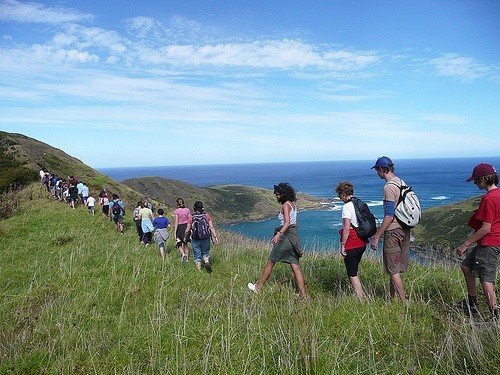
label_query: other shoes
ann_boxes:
[182,254,187,262]
[145,243,149,247]
[457,299,479,315]
[205,262,212,273]
[248,283,256,293]
[120,231,124,235]
[140,240,144,244]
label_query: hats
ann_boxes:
[465,163,497,183]
[370,156,393,170]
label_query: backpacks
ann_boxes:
[191,212,211,239]
[384,181,421,227]
[111,200,123,216]
[346,196,376,240]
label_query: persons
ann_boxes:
[456,163,500,322]
[144,197,154,213]
[174,198,191,262]
[40,168,68,202]
[370,156,410,303]
[70,176,77,185]
[335,181,370,298]
[139,202,153,247]
[100,187,111,221]
[108,194,125,235]
[134,202,144,243]
[77,181,83,194]
[86,193,96,216]
[153,209,170,257]
[247,182,305,297]
[183,201,218,274]
[82,183,88,207]
[69,183,78,209]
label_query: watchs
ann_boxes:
[279,231,283,237]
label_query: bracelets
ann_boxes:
[341,242,346,245]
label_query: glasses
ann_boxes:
[337,193,340,198]
[276,193,282,196]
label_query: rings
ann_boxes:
[276,241,277,242]
